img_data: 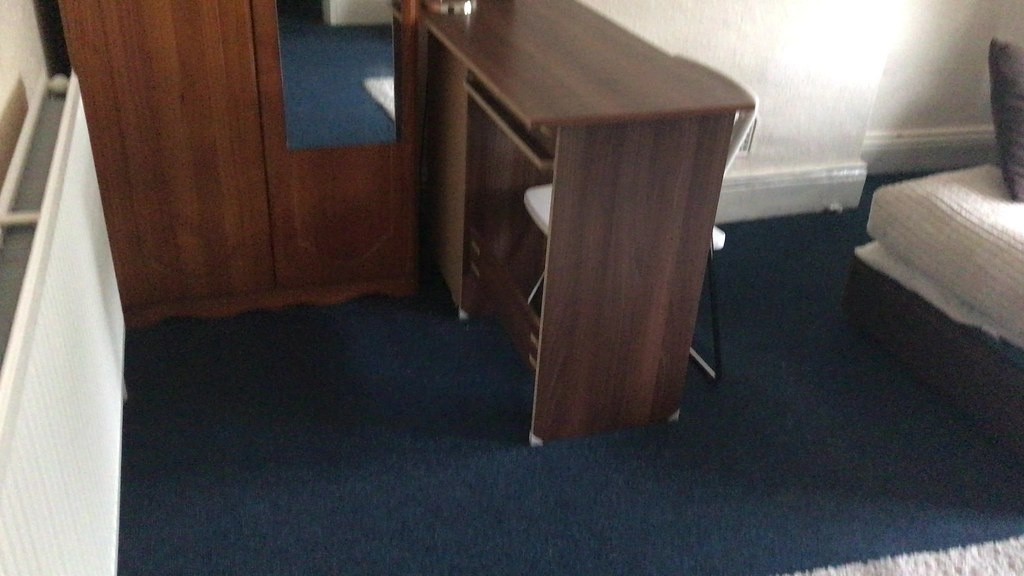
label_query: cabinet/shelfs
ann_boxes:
[59,0,418,322]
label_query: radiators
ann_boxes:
[0,69,126,576]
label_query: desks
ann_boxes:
[417,0,754,449]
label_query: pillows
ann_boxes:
[989,37,1024,201]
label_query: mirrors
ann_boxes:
[275,0,403,151]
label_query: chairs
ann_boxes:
[525,55,757,385]
